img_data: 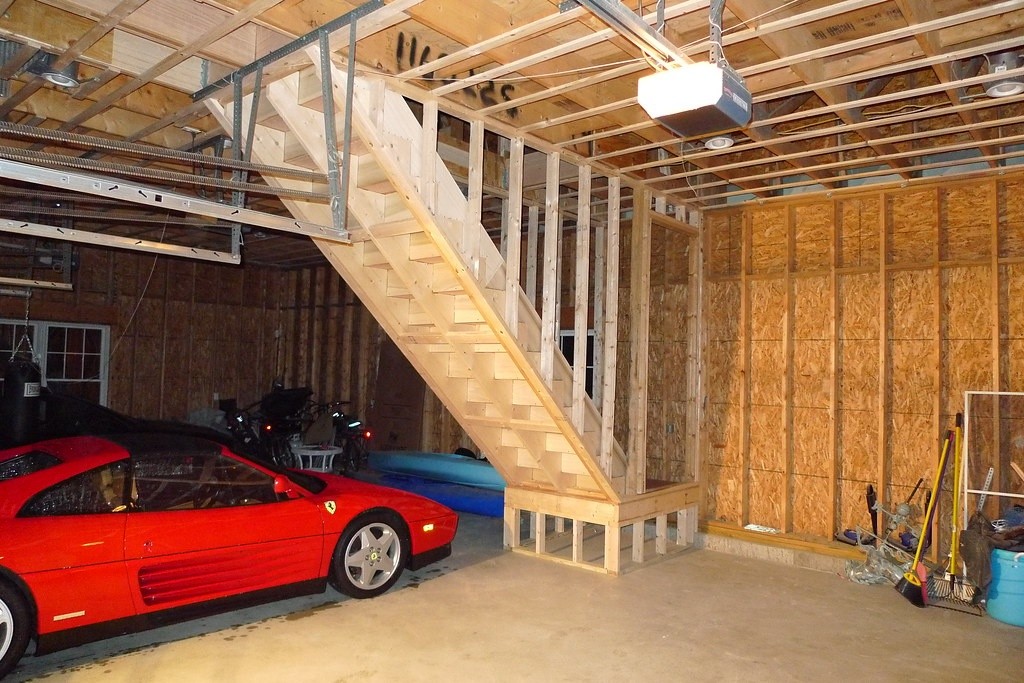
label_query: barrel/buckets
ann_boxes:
[986,549,1024,628]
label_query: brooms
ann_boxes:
[893,412,982,608]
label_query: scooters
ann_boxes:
[225,382,371,468]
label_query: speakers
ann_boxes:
[637,61,752,138]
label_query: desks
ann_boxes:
[292,446,343,473]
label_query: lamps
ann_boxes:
[705,134,733,149]
[980,48,1024,97]
[41,53,79,87]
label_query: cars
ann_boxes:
[0,429,459,682]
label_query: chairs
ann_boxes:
[93,467,116,503]
